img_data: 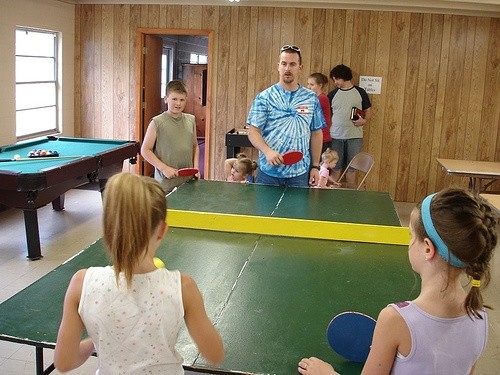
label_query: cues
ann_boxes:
[0,156,81,162]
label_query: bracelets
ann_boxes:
[312,165,320,170]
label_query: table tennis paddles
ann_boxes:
[326,312,377,363]
[273,152,303,166]
[164,168,199,179]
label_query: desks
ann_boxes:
[0,179,422,375]
[435,159,500,193]
[0,135,141,261]
[479,194,500,213]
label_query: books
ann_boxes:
[350,107,366,121]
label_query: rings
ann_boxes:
[304,363,308,370]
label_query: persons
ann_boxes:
[306,73,332,175]
[298,187,497,375]
[53,171,224,375]
[326,64,372,190]
[141,80,200,196]
[224,153,258,183]
[245,44,327,189]
[310,148,343,188]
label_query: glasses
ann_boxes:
[280,45,301,54]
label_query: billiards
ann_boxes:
[13,155,20,158]
[29,148,58,156]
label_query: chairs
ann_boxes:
[326,152,376,191]
